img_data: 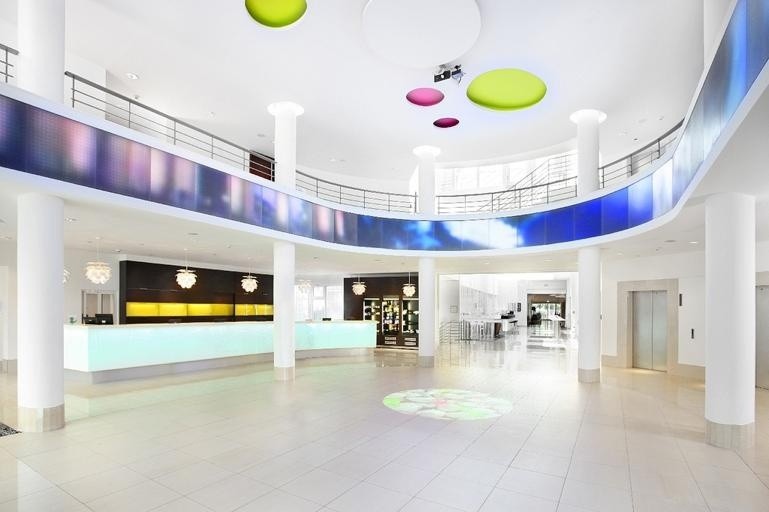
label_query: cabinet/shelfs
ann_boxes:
[363,294,418,347]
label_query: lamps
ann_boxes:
[83,237,113,284]
[239,262,260,293]
[352,266,367,296]
[297,278,312,293]
[174,247,198,290]
[400,268,416,296]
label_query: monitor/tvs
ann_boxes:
[85,317,96,324]
[323,318,331,321]
[95,314,113,324]
